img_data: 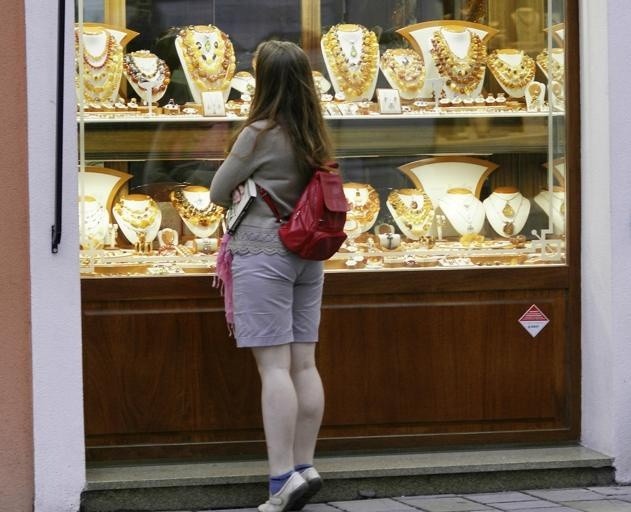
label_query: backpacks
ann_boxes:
[258,117,350,261]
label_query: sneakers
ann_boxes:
[258,466,324,512]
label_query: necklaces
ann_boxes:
[311,25,565,241]
[76,25,256,242]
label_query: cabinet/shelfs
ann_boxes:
[74,1,580,463]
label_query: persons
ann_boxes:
[210,40,335,512]
